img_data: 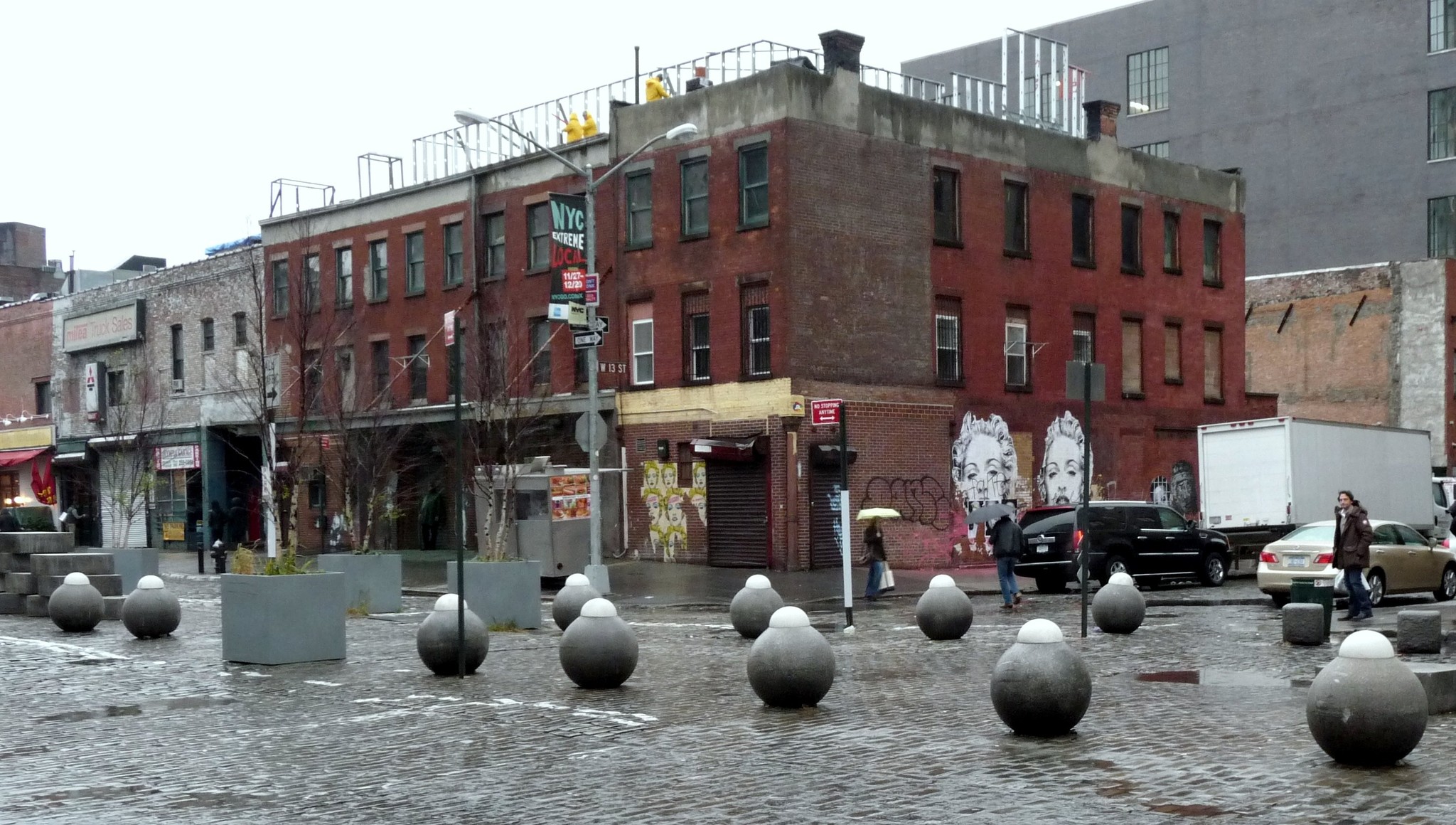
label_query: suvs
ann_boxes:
[1014,500,1233,595]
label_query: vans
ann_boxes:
[0,506,58,532]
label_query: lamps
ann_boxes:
[3,411,50,426]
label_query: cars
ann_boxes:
[1258,520,1456,611]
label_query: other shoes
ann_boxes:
[1337,613,1355,621]
[865,596,877,601]
[1353,611,1373,621]
[1013,596,1022,604]
[999,603,1013,609]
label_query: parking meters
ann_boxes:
[196,520,205,573]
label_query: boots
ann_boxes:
[427,535,438,550]
[420,536,429,551]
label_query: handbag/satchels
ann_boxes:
[858,553,875,565]
[878,564,895,594]
[59,512,69,523]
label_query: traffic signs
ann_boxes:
[318,435,330,450]
[260,353,281,410]
[444,310,455,347]
[811,399,842,425]
[569,315,609,350]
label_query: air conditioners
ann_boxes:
[172,379,186,393]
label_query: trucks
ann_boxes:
[1197,417,1456,570]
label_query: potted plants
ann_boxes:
[318,551,402,613]
[219,536,346,663]
[445,557,543,628]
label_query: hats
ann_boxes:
[231,497,242,505]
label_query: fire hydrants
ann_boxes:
[211,539,228,573]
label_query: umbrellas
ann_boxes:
[963,504,1017,524]
[856,508,901,533]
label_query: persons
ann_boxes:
[1332,491,1375,622]
[562,113,583,143]
[416,479,441,551]
[646,74,670,102]
[988,515,1024,609]
[66,499,86,546]
[209,501,226,539]
[863,516,888,601]
[581,110,598,138]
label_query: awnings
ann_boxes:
[0,448,46,467]
[87,434,139,449]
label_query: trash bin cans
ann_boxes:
[1290,576,1335,643]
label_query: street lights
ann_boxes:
[455,112,698,596]
